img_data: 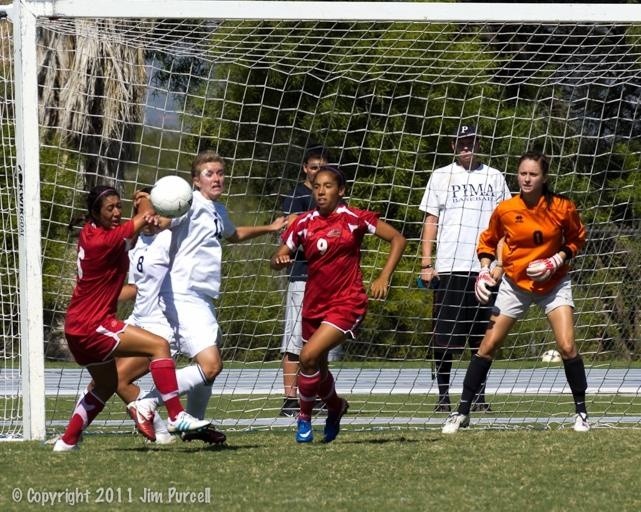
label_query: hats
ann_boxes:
[449,122,481,141]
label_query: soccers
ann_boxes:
[148,174,194,217]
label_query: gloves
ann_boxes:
[475,267,497,305]
[526,252,564,283]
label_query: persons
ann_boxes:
[107,188,176,443]
[440,151,594,436]
[267,167,408,444]
[420,124,517,415]
[279,146,335,420]
[124,148,290,444]
[50,186,212,454]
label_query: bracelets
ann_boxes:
[420,263,433,270]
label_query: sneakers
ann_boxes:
[52,437,75,453]
[181,423,227,446]
[167,411,211,434]
[295,419,314,442]
[148,432,176,444]
[323,399,349,443]
[311,398,326,417]
[126,399,156,442]
[279,395,300,417]
[442,412,470,434]
[573,412,591,433]
[473,403,492,412]
[434,401,451,412]
